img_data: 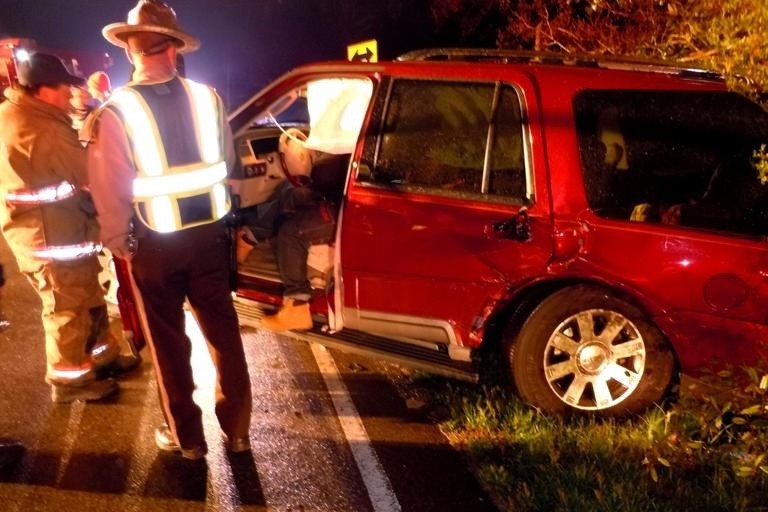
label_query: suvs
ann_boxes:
[228,49,767,427]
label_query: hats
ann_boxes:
[86,70,110,94]
[16,52,85,88]
[101,0,201,55]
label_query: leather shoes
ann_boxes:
[153,424,208,461]
[50,375,118,403]
[221,431,252,454]
[94,352,141,377]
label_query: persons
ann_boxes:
[1,54,142,403]
[83,1,255,459]
[67,85,95,136]
[86,71,114,105]
[231,176,346,332]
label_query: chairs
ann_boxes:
[238,240,335,294]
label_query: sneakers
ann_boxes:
[234,229,254,264]
[260,296,314,332]
